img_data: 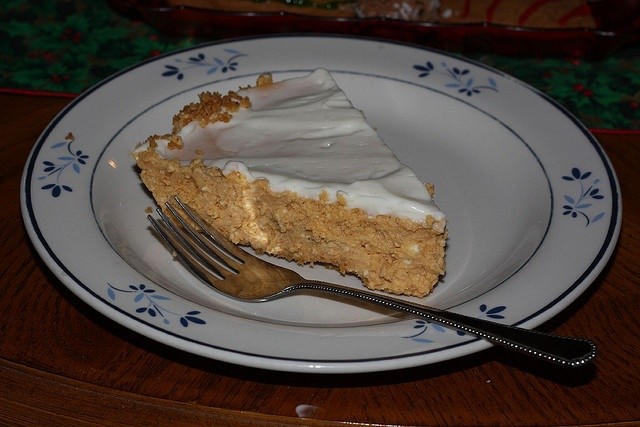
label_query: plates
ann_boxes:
[17,33,623,377]
[141,0,620,59]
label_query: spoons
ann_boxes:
[147,196,597,367]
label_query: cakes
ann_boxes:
[130,69,450,298]
